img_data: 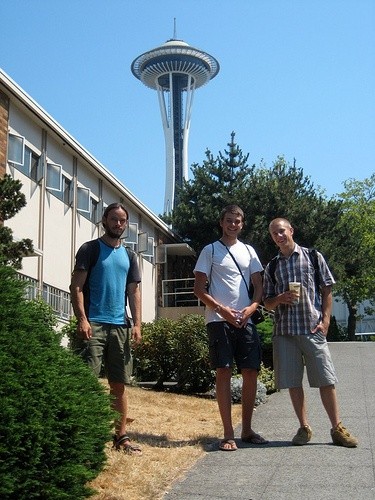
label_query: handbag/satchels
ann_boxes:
[251,309,265,325]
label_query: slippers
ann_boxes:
[219,439,238,451]
[241,434,268,444]
[113,435,141,452]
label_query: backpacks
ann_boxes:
[70,240,135,325]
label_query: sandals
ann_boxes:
[331,421,358,447]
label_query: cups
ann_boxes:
[289,282,301,305]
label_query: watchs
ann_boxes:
[214,305,222,312]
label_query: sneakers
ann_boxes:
[292,425,312,445]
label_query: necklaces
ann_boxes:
[101,237,120,253]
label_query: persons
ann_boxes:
[193,205,267,450]
[263,218,359,447]
[69,203,143,455]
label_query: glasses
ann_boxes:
[106,217,127,223]
